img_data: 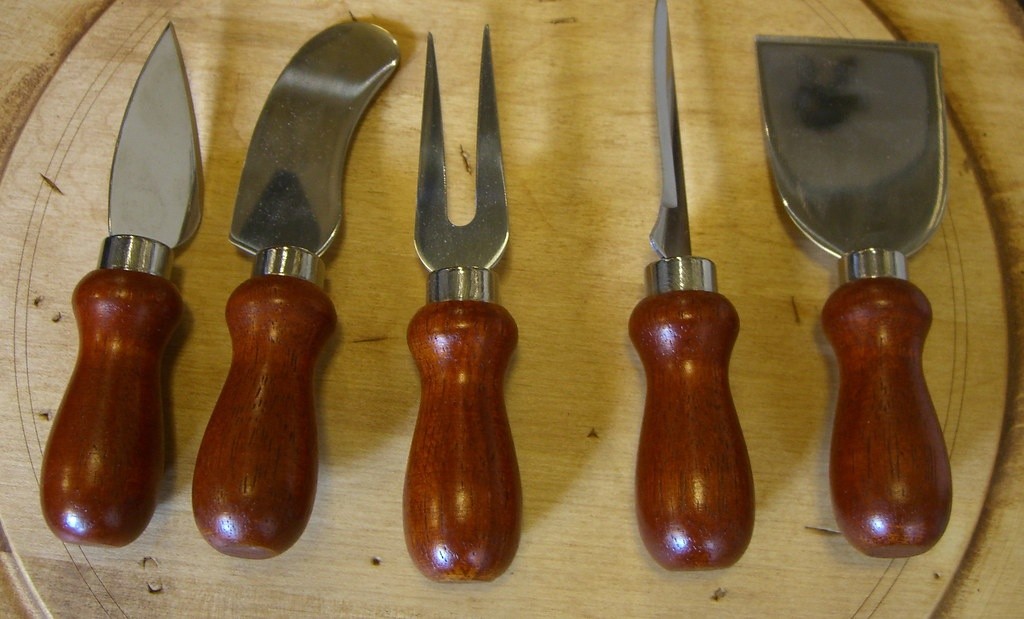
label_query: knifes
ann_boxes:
[38,21,211,550]
[628,0,758,574]
